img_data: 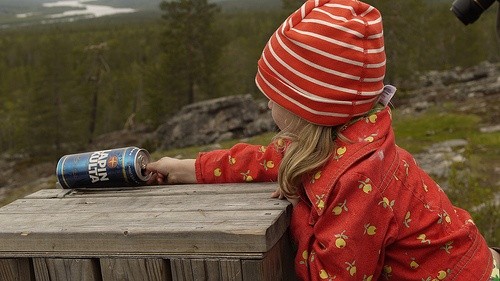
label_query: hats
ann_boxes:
[256,0,386,126]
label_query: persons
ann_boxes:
[142,0,500,281]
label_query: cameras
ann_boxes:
[449,0,497,27]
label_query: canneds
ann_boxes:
[56,146,154,189]
